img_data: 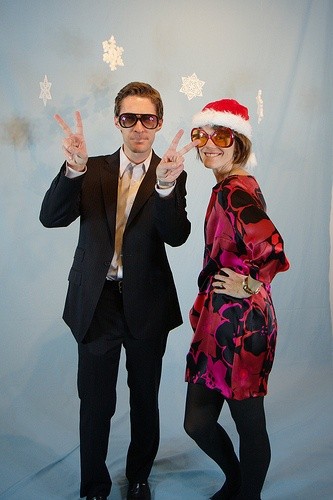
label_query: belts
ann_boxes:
[104,279,123,294]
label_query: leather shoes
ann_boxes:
[128,481,151,500]
[86,493,108,500]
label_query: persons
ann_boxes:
[39,81,202,500]
[184,99,289,500]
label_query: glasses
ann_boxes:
[116,113,159,129]
[191,128,236,148]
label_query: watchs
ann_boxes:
[242,277,261,295]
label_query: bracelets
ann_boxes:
[156,179,175,186]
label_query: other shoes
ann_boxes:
[210,475,241,500]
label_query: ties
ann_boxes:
[115,163,135,266]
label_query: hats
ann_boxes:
[193,99,251,140]
